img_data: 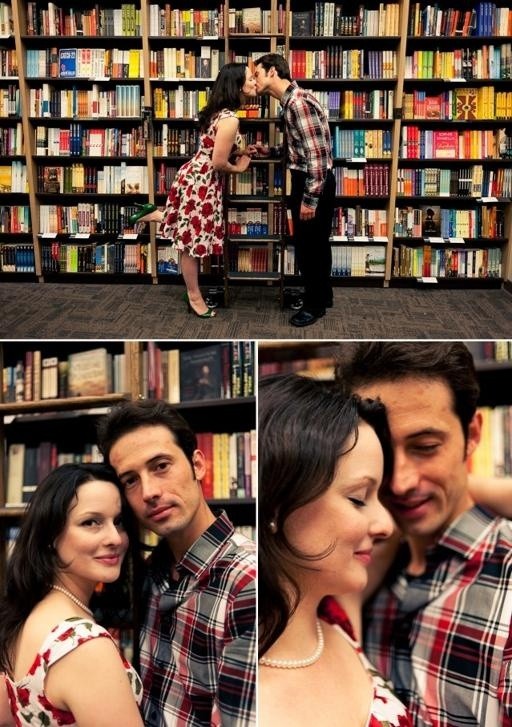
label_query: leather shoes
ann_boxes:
[289,293,335,328]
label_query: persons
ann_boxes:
[253,52,338,331]
[1,462,147,727]
[331,342,512,727]
[100,394,255,727]
[127,61,257,320]
[256,373,512,727]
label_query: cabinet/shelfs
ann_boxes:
[0,395,254,519]
[0,2,512,288]
[218,156,285,309]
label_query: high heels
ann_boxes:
[181,291,218,319]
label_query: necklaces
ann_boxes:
[48,585,96,618]
[258,616,325,670]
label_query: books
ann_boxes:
[260,343,512,477]
[1,341,255,672]
[0,1,511,287]
